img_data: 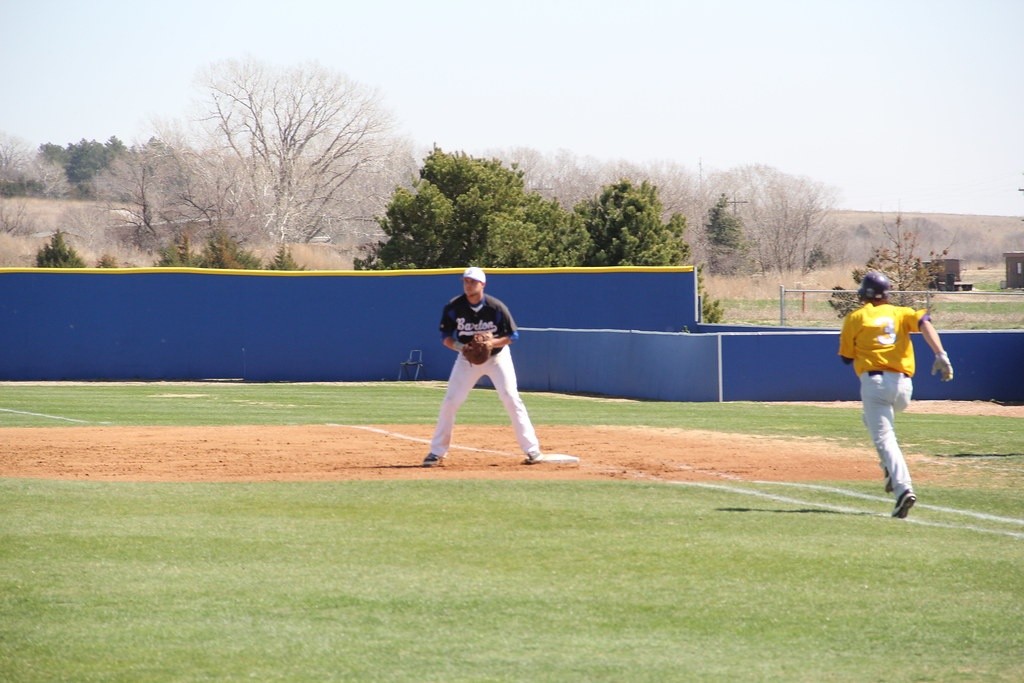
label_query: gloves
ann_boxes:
[931,353,954,381]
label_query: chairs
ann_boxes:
[397,350,424,381]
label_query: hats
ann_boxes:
[463,267,486,284]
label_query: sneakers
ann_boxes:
[423,454,442,464]
[892,488,916,517]
[884,467,891,492]
[527,450,542,464]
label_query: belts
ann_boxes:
[868,369,908,378]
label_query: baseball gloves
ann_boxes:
[463,332,492,365]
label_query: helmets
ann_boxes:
[859,272,891,299]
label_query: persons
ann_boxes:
[838,273,953,518]
[423,267,544,465]
